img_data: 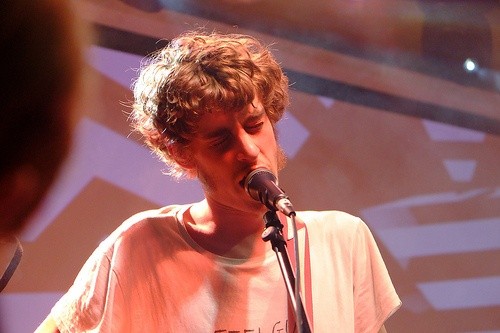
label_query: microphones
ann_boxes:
[244,168,296,219]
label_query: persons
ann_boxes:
[22,30,402,333]
